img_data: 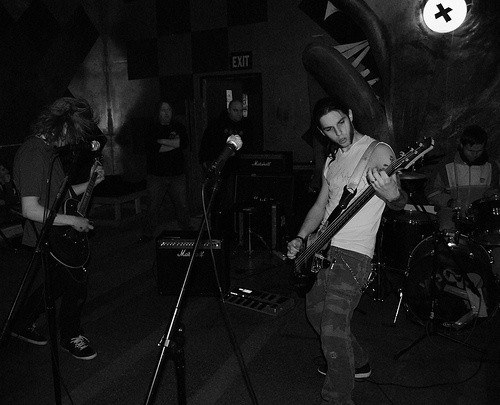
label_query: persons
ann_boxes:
[199,100,263,155]
[132,100,195,245]
[425,127,500,218]
[13,98,105,360]
[286,95,408,405]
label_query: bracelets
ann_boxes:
[295,235,304,243]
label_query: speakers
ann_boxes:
[154,230,231,297]
[234,170,302,239]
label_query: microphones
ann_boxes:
[56,140,101,153]
[204,134,243,185]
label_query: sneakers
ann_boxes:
[11,322,48,346]
[61,334,97,360]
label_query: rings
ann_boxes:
[371,178,377,184]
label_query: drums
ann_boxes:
[384,210,440,273]
[401,231,500,335]
[471,195,500,248]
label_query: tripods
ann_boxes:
[361,183,487,361]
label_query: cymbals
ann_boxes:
[397,172,426,179]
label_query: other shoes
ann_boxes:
[317,363,371,379]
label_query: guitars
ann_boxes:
[290,134,427,283]
[41,153,101,269]
